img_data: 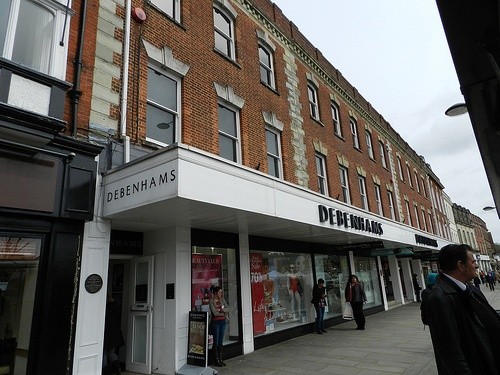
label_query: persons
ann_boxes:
[345,275,368,330]
[420,243,500,375]
[287,264,304,316]
[311,278,328,335]
[209,286,230,367]
[474,269,500,291]
[412,274,422,303]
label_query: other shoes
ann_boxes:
[322,329,326,332]
[317,331,322,334]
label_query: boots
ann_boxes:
[211,344,223,367]
[218,344,226,366]
